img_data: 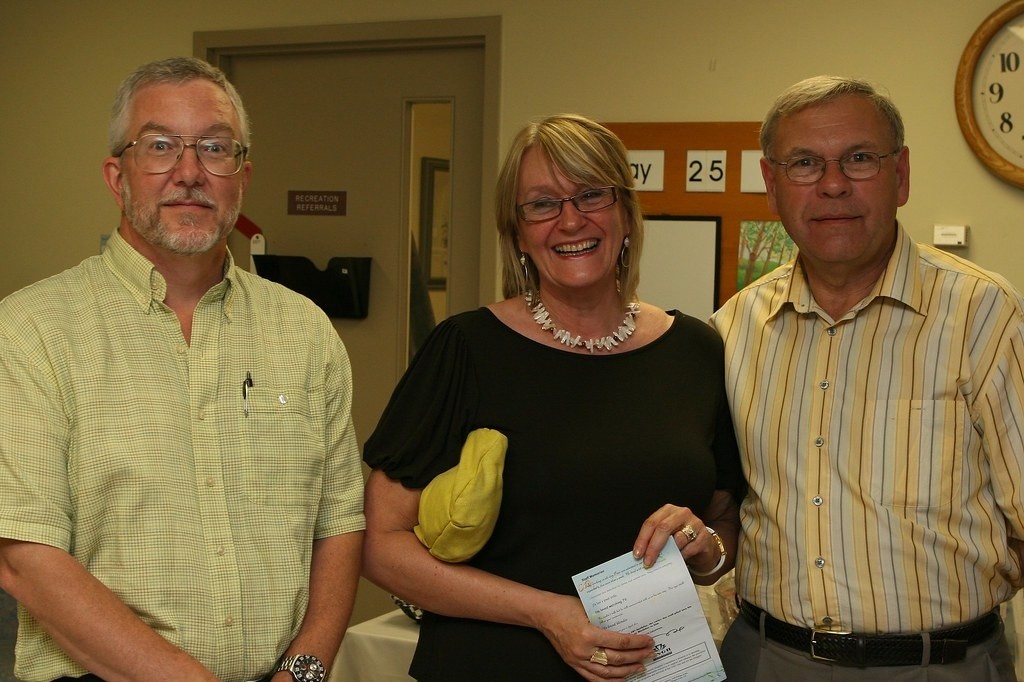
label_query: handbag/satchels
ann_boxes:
[391,428,507,621]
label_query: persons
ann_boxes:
[707,75,1024,682]
[0,54,367,682]
[360,112,751,682]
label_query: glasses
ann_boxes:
[517,186,621,223]
[117,134,248,176]
[768,151,898,184]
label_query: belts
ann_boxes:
[738,599,1002,667]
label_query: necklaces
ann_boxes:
[524,290,640,352]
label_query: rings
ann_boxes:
[590,647,608,666]
[681,524,697,543]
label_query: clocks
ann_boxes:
[955,0,1024,189]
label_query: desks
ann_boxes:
[329,607,418,682]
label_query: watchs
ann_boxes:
[277,654,329,682]
[686,527,728,576]
